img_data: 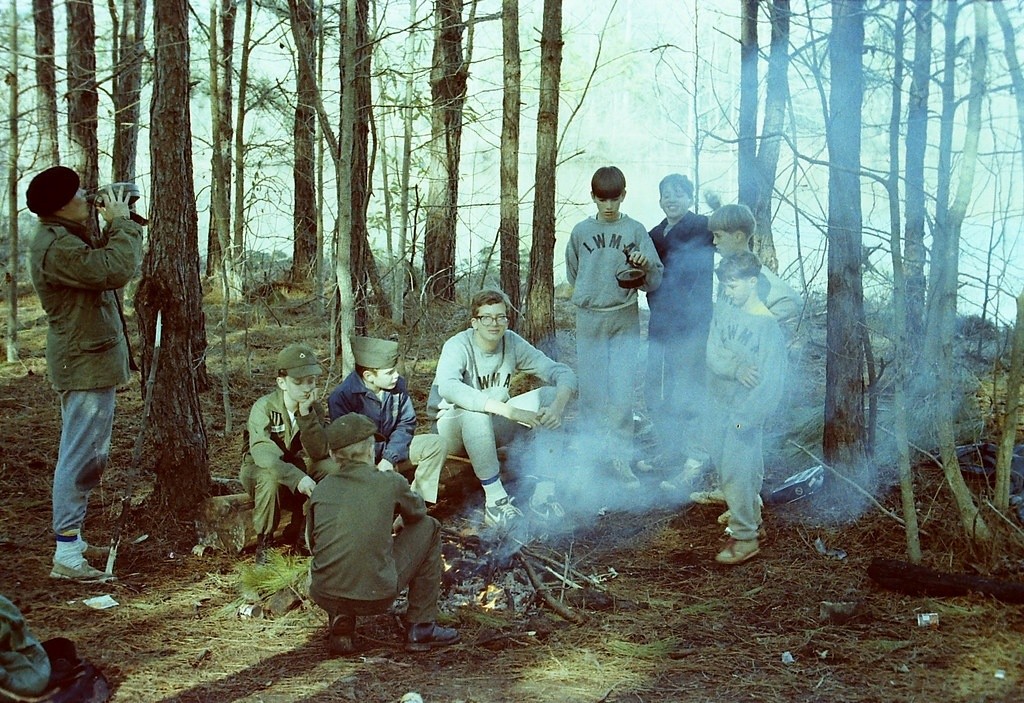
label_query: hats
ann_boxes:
[26,167,80,218]
[325,412,377,450]
[348,337,401,368]
[277,344,322,379]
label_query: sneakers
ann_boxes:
[637,454,680,473]
[756,526,766,542]
[608,460,640,490]
[690,489,727,505]
[529,493,565,520]
[718,510,763,524]
[49,557,118,583]
[715,539,760,564]
[659,464,711,491]
[485,496,525,533]
[82,544,110,560]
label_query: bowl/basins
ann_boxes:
[617,267,647,289]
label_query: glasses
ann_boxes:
[474,315,510,326]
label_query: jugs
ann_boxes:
[87,182,139,206]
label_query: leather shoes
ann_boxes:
[405,622,461,650]
[328,613,355,654]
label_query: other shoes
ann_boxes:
[283,524,305,540]
[256,531,273,566]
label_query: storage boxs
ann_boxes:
[772,465,824,504]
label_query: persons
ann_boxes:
[706,250,787,563]
[26,167,143,581]
[690,204,805,523]
[636,174,724,492]
[240,346,345,566]
[305,412,463,652]
[427,290,579,534]
[565,166,664,489]
[328,335,449,504]
